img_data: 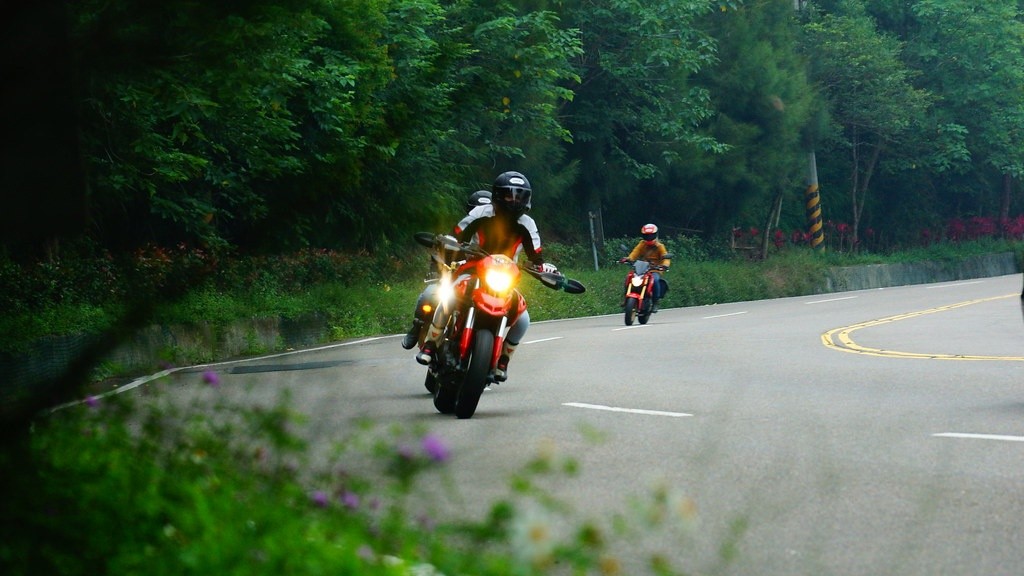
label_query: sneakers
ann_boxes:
[494,354,510,382]
[651,303,658,312]
[401,318,426,349]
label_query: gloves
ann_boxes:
[662,266,669,272]
[541,262,558,274]
[444,234,460,252]
[619,258,626,264]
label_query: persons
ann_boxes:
[402,172,562,382]
[621,223,670,313]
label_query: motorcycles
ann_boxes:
[616,252,674,327]
[412,231,587,420]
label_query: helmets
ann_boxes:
[466,190,493,207]
[641,223,658,247]
[491,171,532,218]
